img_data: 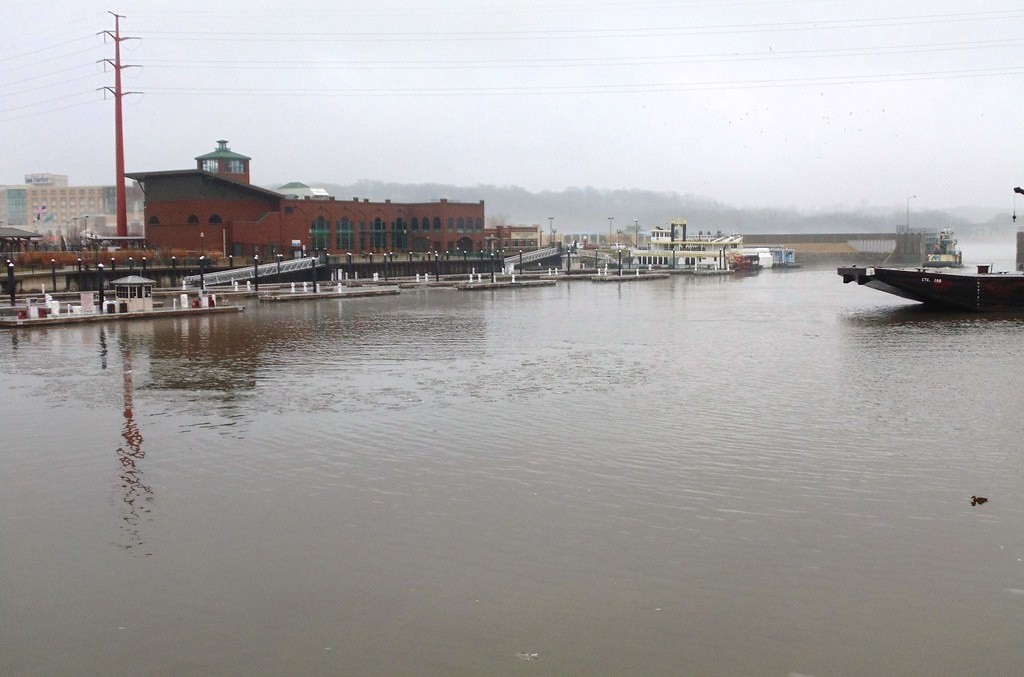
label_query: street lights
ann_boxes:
[200,232,204,256]
[548,217,554,242]
[73,218,78,238]
[634,219,637,250]
[608,217,613,246]
[66,222,69,235]
[907,196,916,233]
[85,216,88,238]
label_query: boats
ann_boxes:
[922,228,963,268]
[837,265,1024,311]
